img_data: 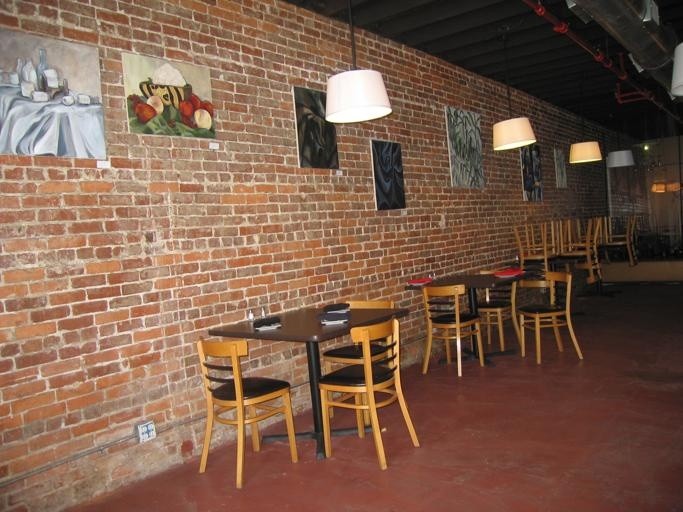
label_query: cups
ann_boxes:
[61,95,75,105]
[21,81,35,98]
[31,90,49,102]
[0,68,20,85]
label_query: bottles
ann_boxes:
[15,56,24,81]
[63,78,69,96]
[21,57,38,91]
[36,47,49,92]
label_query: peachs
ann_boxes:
[146,96,164,116]
[194,109,212,130]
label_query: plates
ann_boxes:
[407,282,429,287]
[327,308,350,314]
[320,318,349,325]
[254,321,282,332]
[494,273,521,278]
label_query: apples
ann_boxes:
[135,103,157,124]
[189,93,201,111]
[201,101,213,118]
[180,100,193,118]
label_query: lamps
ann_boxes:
[608,104,635,168]
[569,77,603,164]
[493,33,537,151]
[651,179,680,200]
[671,41,683,97]
[324,0,392,123]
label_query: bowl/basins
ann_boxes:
[139,81,193,111]
